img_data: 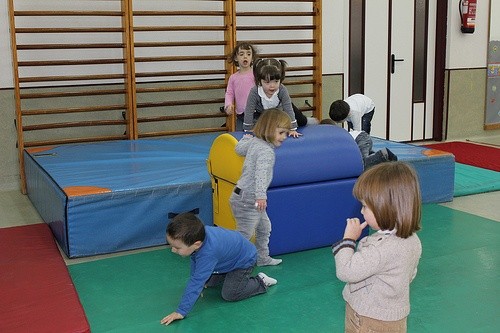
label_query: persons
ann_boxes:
[242,57,307,138]
[160,211,278,326]
[329,94,376,134]
[229,107,293,267]
[319,119,398,171]
[331,160,423,333]
[224,40,259,132]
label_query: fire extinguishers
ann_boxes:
[458,0,477,33]
